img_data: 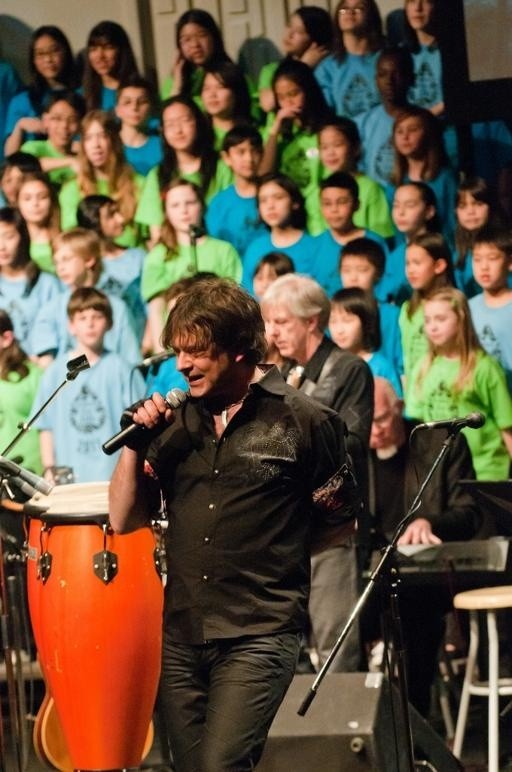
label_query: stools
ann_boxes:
[452,586,512,772]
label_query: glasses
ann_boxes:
[337,5,366,15]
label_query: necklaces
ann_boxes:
[211,367,265,429]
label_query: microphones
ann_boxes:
[190,221,202,239]
[102,388,186,458]
[417,410,487,429]
[2,456,54,500]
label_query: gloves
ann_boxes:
[120,394,173,452]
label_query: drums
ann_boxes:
[25,483,168,772]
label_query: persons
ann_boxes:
[297,168,391,299]
[161,8,254,121]
[3,25,76,158]
[252,250,295,299]
[348,44,460,189]
[0,151,45,208]
[145,273,215,399]
[75,19,145,111]
[360,377,479,720]
[0,206,62,356]
[20,87,87,178]
[398,232,459,395]
[313,0,384,119]
[133,93,235,251]
[28,286,146,487]
[58,109,149,251]
[405,287,512,481]
[255,58,336,189]
[197,57,252,153]
[305,116,395,238]
[108,279,361,771]
[240,170,316,303]
[114,76,161,176]
[142,177,243,357]
[328,286,402,401]
[260,274,375,672]
[384,180,463,299]
[75,193,146,314]
[402,1,461,117]
[386,105,464,243]
[0,60,25,163]
[449,173,512,298]
[465,223,512,381]
[258,6,336,137]
[12,169,62,279]
[324,236,405,392]
[204,122,267,258]
[31,228,143,371]
[1,308,46,476]
